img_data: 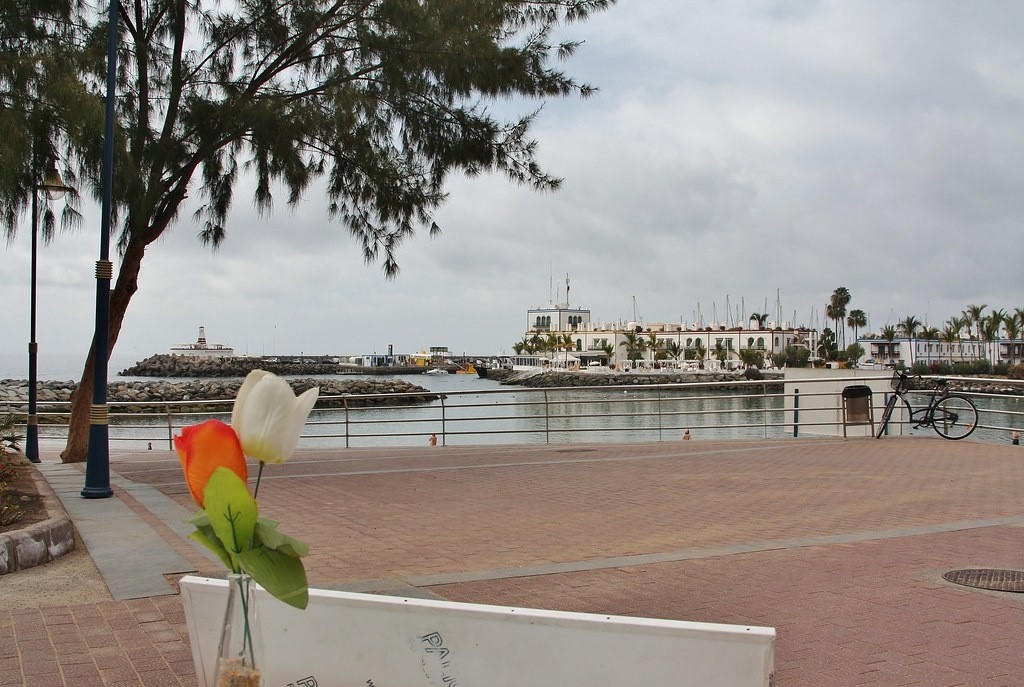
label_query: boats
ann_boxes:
[169,326,234,356]
[474,359,513,378]
[336,368,364,375]
[456,363,477,374]
[427,368,448,374]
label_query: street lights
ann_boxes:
[25,185,76,464]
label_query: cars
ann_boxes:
[303,359,317,364]
[292,359,299,362]
[321,359,334,364]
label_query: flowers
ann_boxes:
[175,368,321,670]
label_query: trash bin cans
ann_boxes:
[845,385,869,422]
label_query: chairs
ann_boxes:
[842,385,875,439]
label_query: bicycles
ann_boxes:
[876,362,979,441]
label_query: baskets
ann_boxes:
[891,371,915,394]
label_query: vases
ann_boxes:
[212,572,260,687]
